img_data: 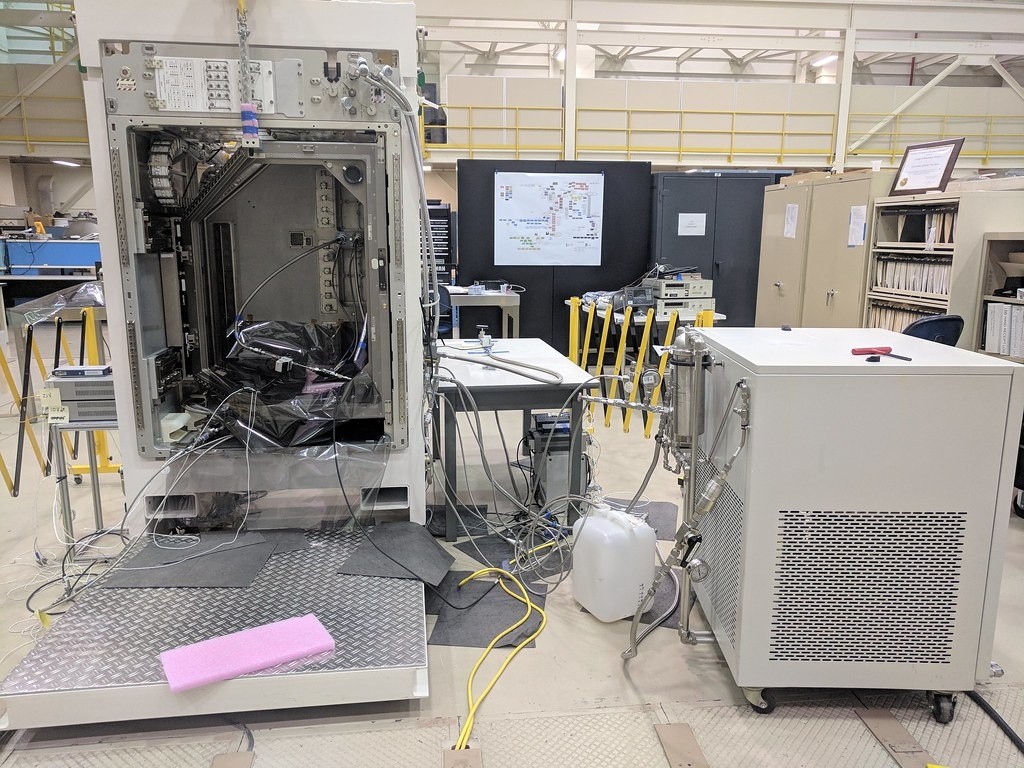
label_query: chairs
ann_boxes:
[901,315,964,348]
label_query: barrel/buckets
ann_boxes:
[573,504,656,622]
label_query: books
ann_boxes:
[860,211,957,335]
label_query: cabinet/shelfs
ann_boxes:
[646,172,792,327]
[753,168,900,328]
[971,231,1024,365]
[860,191,1024,328]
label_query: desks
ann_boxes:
[582,305,727,376]
[450,289,520,339]
[431,338,600,542]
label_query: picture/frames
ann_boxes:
[888,137,966,197]
